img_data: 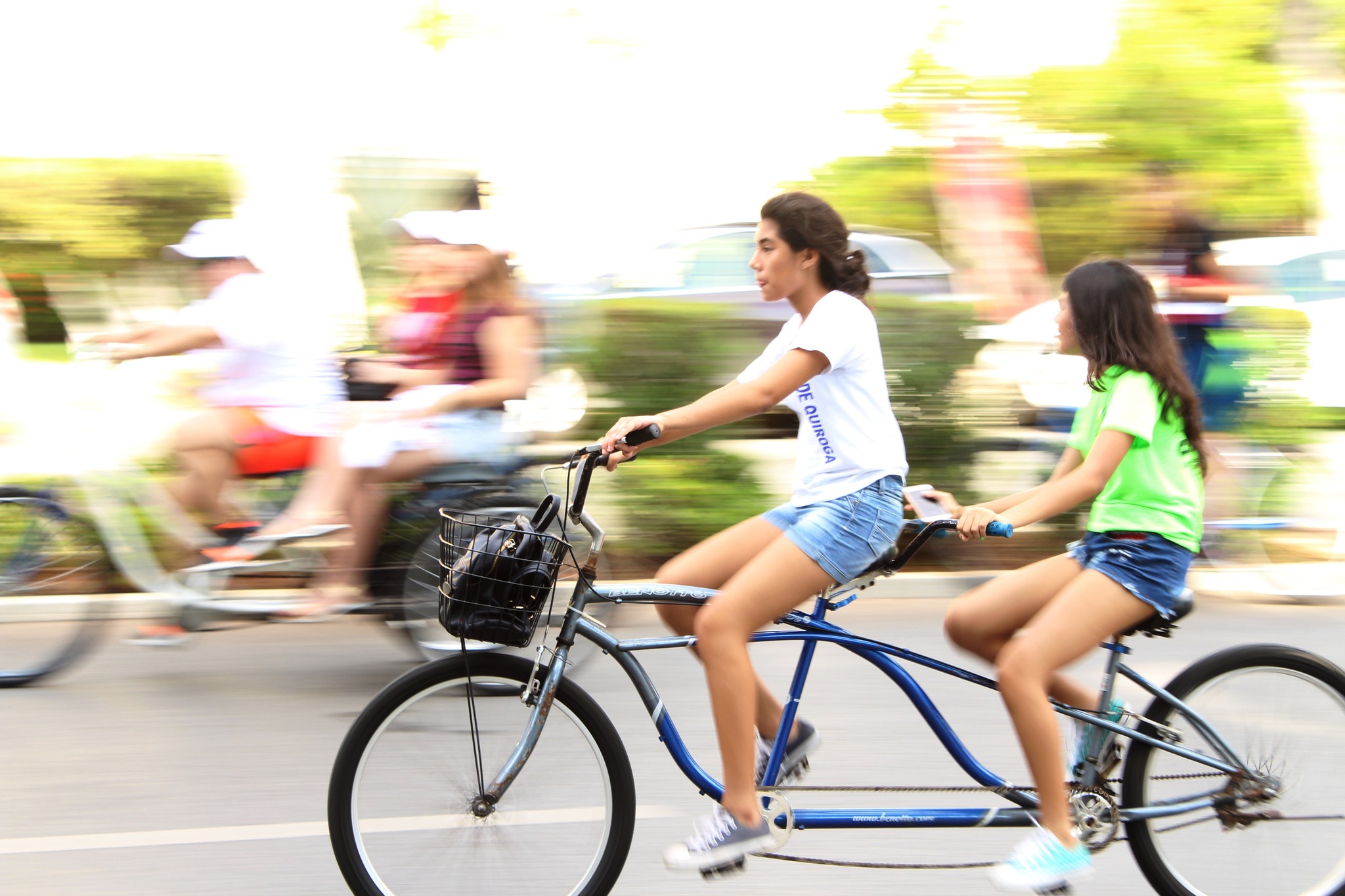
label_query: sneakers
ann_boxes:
[1069,690,1131,779]
[662,808,774,873]
[989,825,1096,894]
[752,718,822,790]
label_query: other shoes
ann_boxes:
[122,620,190,647]
[247,508,357,550]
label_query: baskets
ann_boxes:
[436,507,572,648]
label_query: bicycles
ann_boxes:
[0,341,618,695]
[326,423,1345,894]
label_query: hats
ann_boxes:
[437,206,509,257]
[376,211,455,240]
[160,218,245,262]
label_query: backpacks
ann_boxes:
[438,492,561,647]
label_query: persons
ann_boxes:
[602,191,911,874]
[902,259,1211,892]
[77,210,546,648]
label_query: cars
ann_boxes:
[666,223,957,314]
